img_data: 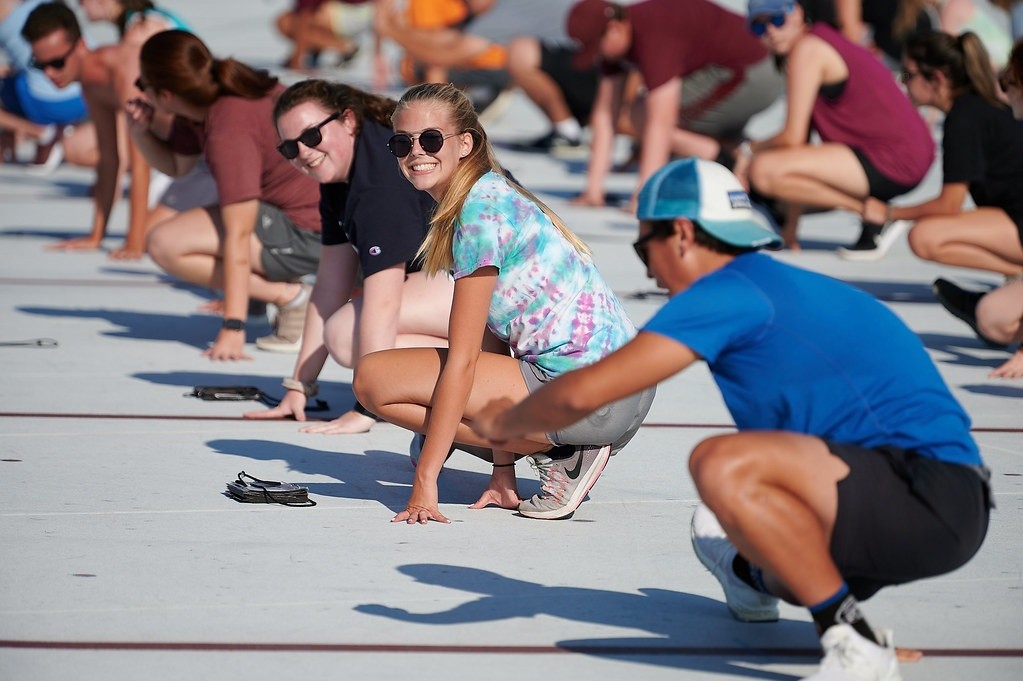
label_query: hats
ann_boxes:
[566,0,615,70]
[747,0,797,32]
[636,157,785,251]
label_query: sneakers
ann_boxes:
[410,433,456,471]
[713,149,746,178]
[520,443,612,520]
[532,130,581,150]
[799,624,902,681]
[833,218,914,262]
[612,143,642,174]
[932,277,1006,348]
[690,502,780,621]
[247,303,269,326]
[257,281,314,353]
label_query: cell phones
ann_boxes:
[195,386,259,400]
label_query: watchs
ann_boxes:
[222,320,246,330]
[281,378,319,397]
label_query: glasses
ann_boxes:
[385,129,465,157]
[751,13,785,35]
[900,67,917,83]
[31,42,76,70]
[134,78,152,91]
[999,77,1018,93]
[633,226,673,267]
[275,111,341,160]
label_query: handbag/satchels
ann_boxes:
[222,471,317,508]
[193,383,330,420]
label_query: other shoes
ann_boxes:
[29,123,76,173]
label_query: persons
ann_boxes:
[244,79,511,436]
[351,82,656,524]
[277,0,1023,380]
[0,1,321,360]
[472,156,995,681]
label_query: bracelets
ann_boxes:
[492,463,516,467]
